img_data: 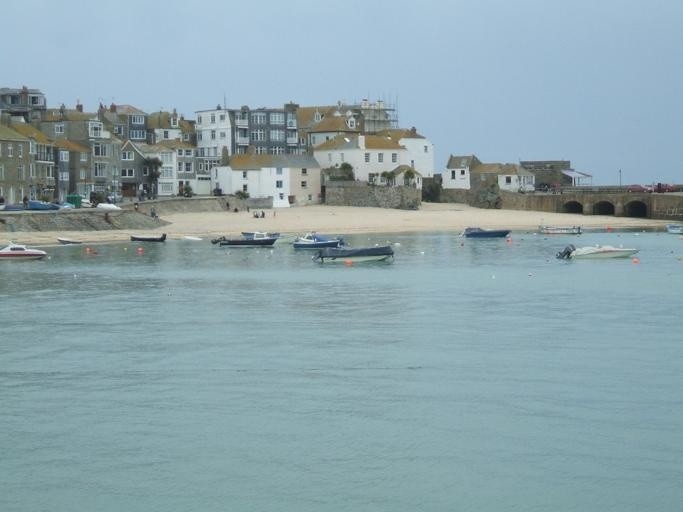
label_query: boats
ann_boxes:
[461,227,513,238]
[58,237,82,244]
[210,231,279,248]
[538,224,584,235]
[130,232,166,242]
[666,224,683,234]
[557,244,640,259]
[293,233,395,263]
[0,244,47,260]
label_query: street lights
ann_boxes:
[619,168,623,189]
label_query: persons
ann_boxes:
[23,195,30,208]
[519,185,525,194]
[227,201,265,219]
[545,183,564,195]
[149,205,155,218]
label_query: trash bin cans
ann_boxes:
[66,195,82,208]
[213,189,222,196]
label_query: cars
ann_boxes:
[109,192,122,201]
[627,184,675,192]
